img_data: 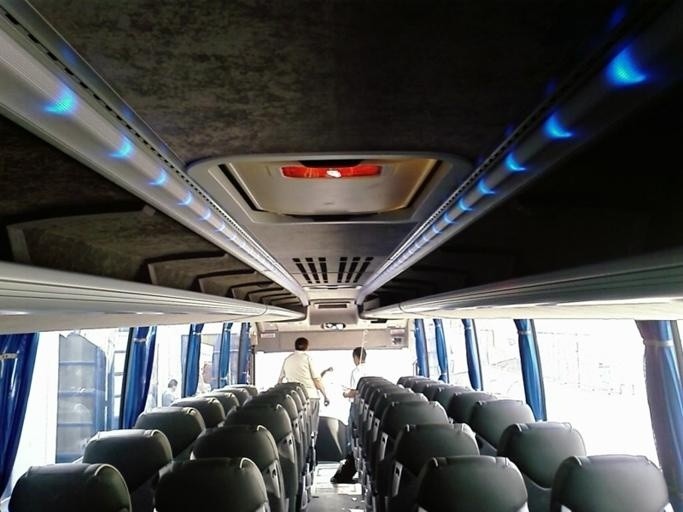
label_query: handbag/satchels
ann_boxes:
[330,451,356,483]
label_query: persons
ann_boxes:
[160,377,178,406]
[275,336,331,406]
[338,346,371,464]
[194,372,211,396]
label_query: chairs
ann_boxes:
[7,381,318,512]
[348,375,672,512]
